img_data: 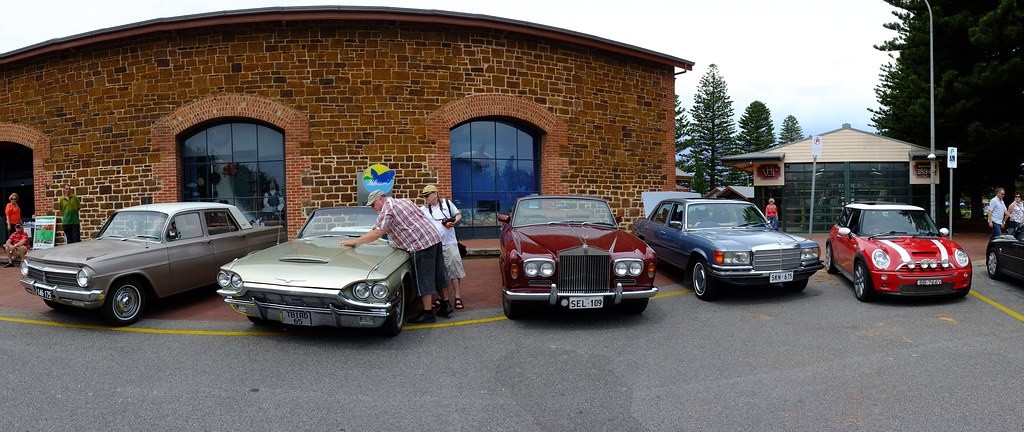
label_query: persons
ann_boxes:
[987,187,1014,239]
[1001,191,1024,230]
[419,185,466,310]
[340,190,454,324]
[263,182,285,219]
[3,193,31,268]
[766,197,779,229]
[58,185,82,244]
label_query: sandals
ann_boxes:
[432,296,441,308]
[454,298,464,309]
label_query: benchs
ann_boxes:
[206,223,235,234]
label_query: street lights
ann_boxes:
[927,153,936,232]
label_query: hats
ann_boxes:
[418,185,439,196]
[366,190,386,205]
[15,223,22,228]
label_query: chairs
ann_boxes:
[520,208,548,224]
[566,211,594,221]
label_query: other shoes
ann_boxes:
[4,262,13,268]
[409,310,436,324]
[436,300,453,316]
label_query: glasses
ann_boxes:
[11,198,17,200]
[1015,196,1020,198]
[1001,193,1005,196]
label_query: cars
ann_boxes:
[496,195,659,318]
[813,196,844,227]
[986,221,1024,286]
[19,202,284,325]
[825,200,973,302]
[217,205,418,334]
[633,192,826,299]
